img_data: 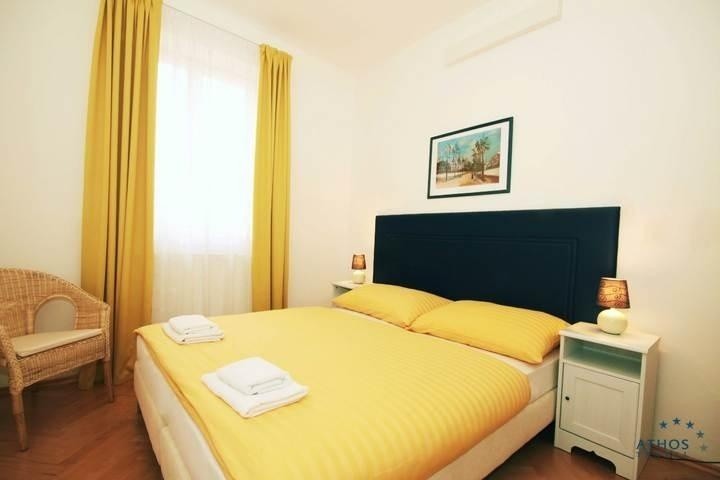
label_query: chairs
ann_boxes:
[1,268,114,451]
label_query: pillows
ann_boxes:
[410,302,571,363]
[333,283,450,328]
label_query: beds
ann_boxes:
[132,207,620,480]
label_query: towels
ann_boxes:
[216,358,286,395]
[163,325,223,343]
[169,314,213,334]
[201,375,307,419]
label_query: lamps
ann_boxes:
[352,254,366,284]
[596,278,630,335]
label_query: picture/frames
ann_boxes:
[427,117,512,198]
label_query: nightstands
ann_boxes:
[332,281,370,290]
[555,322,661,479]
